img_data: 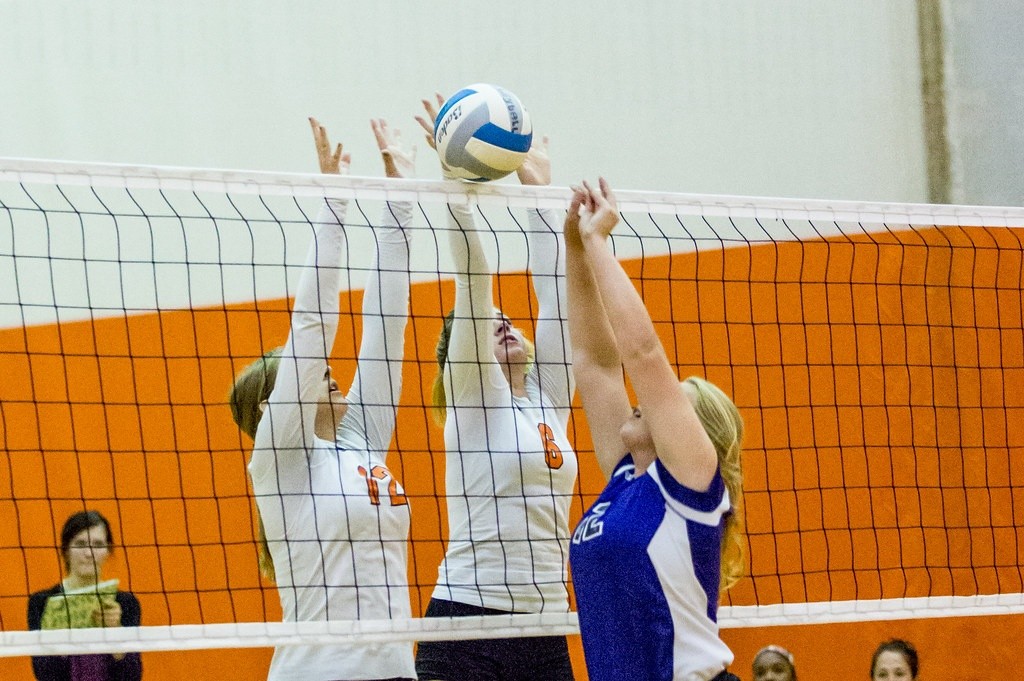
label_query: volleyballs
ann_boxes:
[432,81,536,186]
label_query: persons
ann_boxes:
[27,511,141,681]
[871,639,918,681]
[415,94,579,681]
[230,117,419,681]
[751,645,797,681]
[562,176,744,681]
